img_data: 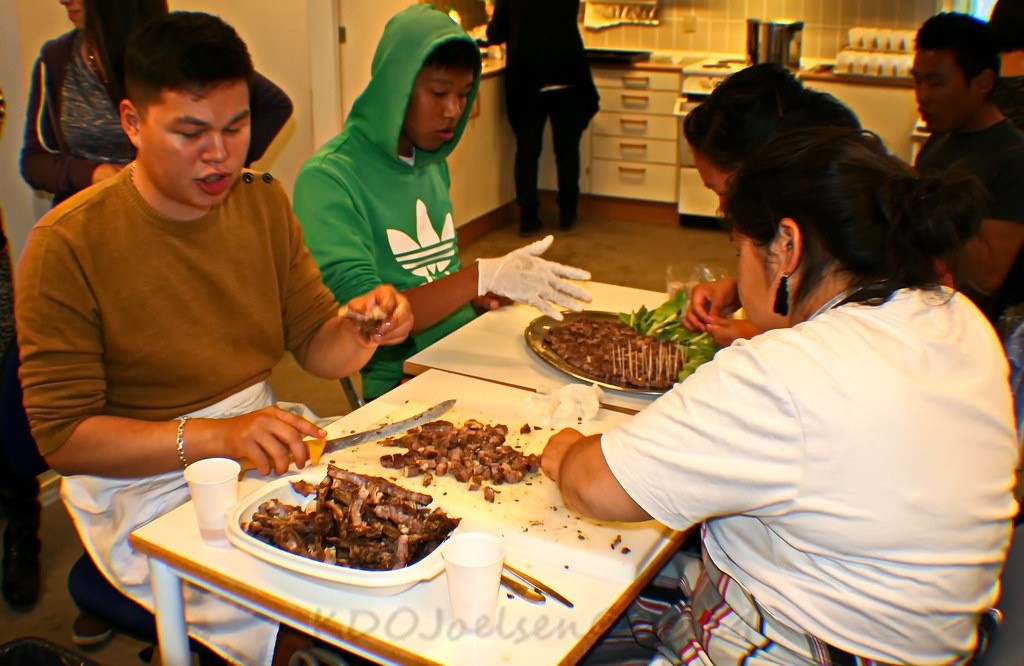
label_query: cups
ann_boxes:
[705,263,745,325]
[441,532,506,635]
[667,261,702,306]
[183,458,240,547]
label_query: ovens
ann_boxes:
[674,77,823,232]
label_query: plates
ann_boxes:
[224,473,465,596]
[525,311,686,396]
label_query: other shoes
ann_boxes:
[72,611,112,646]
[2,547,40,612]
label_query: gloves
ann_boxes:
[476,234,593,321]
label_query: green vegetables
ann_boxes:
[619,290,717,383]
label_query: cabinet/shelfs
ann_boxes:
[447,72,518,229]
[524,67,739,222]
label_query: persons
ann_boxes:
[982,0,1024,125]
[487,0,600,236]
[543,59,1024,666]
[898,10,1024,307]
[289,5,593,404]
[0,0,293,644]
[16,11,414,666]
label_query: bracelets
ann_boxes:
[177,416,190,470]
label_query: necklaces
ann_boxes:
[129,162,136,184]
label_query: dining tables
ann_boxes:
[125,369,695,666]
[402,278,701,414]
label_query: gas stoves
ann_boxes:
[682,53,836,95]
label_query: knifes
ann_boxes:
[233,400,457,481]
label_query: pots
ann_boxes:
[746,18,803,80]
